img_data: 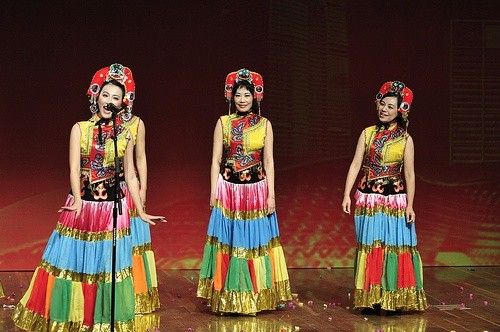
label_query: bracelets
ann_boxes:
[137,210,146,215]
[268,197,276,200]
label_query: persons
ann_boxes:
[14,64,168,332]
[196,69,293,316]
[342,80,429,315]
[88,100,162,316]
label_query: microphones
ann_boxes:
[107,103,120,113]
